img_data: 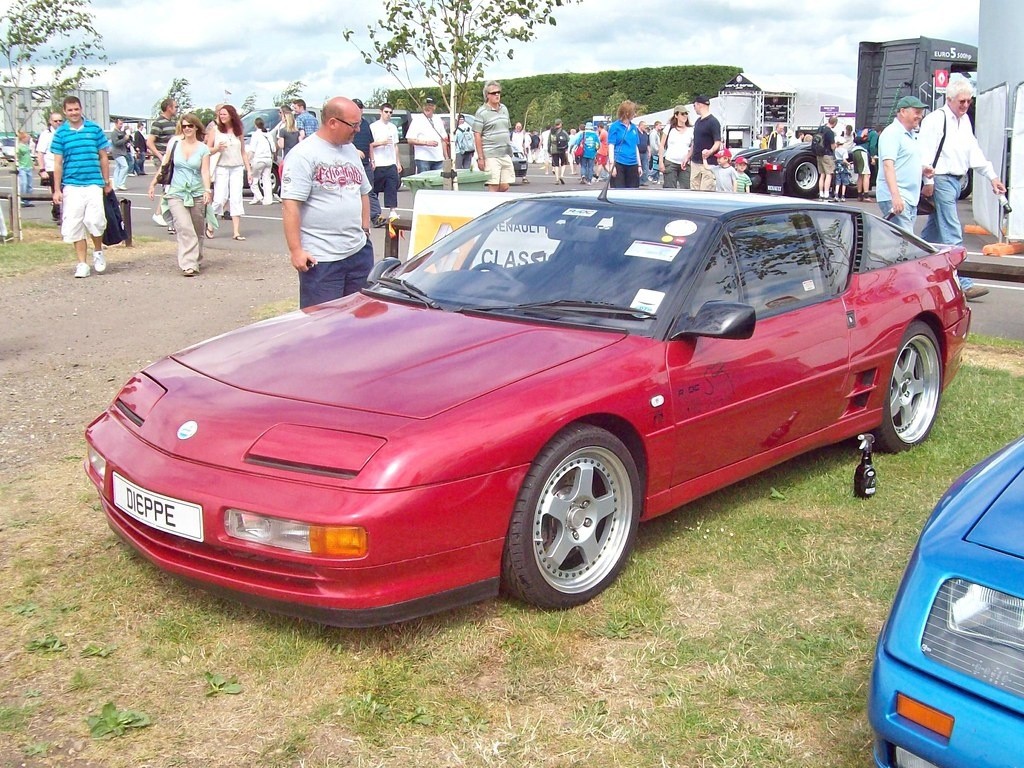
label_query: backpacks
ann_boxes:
[811,128,829,156]
[556,129,568,150]
[100,187,128,246]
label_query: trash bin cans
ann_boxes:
[400,168,492,205]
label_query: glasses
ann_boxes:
[182,124,194,129]
[50,120,62,122]
[357,105,363,109]
[335,118,361,128]
[953,99,973,105]
[383,110,392,114]
[679,111,689,115]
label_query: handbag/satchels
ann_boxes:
[157,140,179,184]
[575,132,585,157]
[652,153,664,172]
[916,181,935,216]
[209,150,221,182]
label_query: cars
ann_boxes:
[102,130,114,159]
[83,185,972,629]
[866,433,1024,768]
[0,137,36,162]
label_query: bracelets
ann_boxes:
[214,146,219,152]
[248,172,253,176]
[205,189,211,193]
[638,165,642,167]
[40,169,45,172]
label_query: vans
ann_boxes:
[240,105,412,195]
[414,112,528,177]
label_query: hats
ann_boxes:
[352,99,366,108]
[585,122,593,129]
[735,157,748,165]
[555,118,562,125]
[424,97,435,104]
[897,97,929,109]
[674,106,690,114]
[714,149,731,157]
[692,97,709,105]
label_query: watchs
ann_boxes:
[363,227,372,235]
[478,157,484,160]
[370,160,375,163]
[105,181,110,186]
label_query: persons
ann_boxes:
[471,79,516,192]
[454,95,881,202]
[918,79,1007,298]
[281,97,374,309]
[876,96,934,238]
[406,96,449,175]
[14,132,32,209]
[35,94,403,278]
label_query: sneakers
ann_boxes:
[75,264,90,279]
[92,247,107,272]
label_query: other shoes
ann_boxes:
[818,193,876,204]
[152,193,281,277]
[128,172,136,177]
[374,218,388,226]
[388,215,400,220]
[52,212,61,225]
[552,169,599,185]
[965,285,990,299]
[118,185,127,191]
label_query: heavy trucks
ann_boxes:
[732,35,979,201]
